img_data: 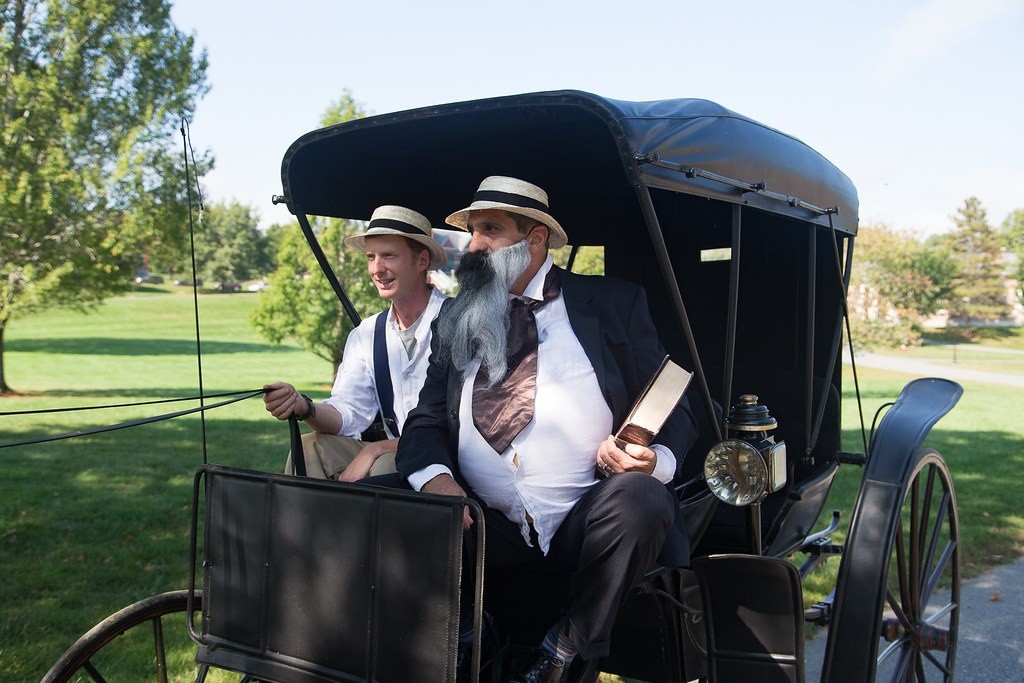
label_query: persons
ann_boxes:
[395,174,688,682]
[263,205,449,483]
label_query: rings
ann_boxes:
[602,463,609,470]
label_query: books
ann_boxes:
[596,355,695,477]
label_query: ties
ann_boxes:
[471,264,563,455]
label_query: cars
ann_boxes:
[174,278,203,287]
[214,280,242,292]
[247,282,271,293]
[135,275,164,285]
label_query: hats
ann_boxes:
[444,177,568,249]
[344,206,446,271]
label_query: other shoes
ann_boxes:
[510,653,569,683]
[455,617,510,683]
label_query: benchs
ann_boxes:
[674,362,830,542]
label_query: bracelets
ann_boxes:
[295,393,317,422]
